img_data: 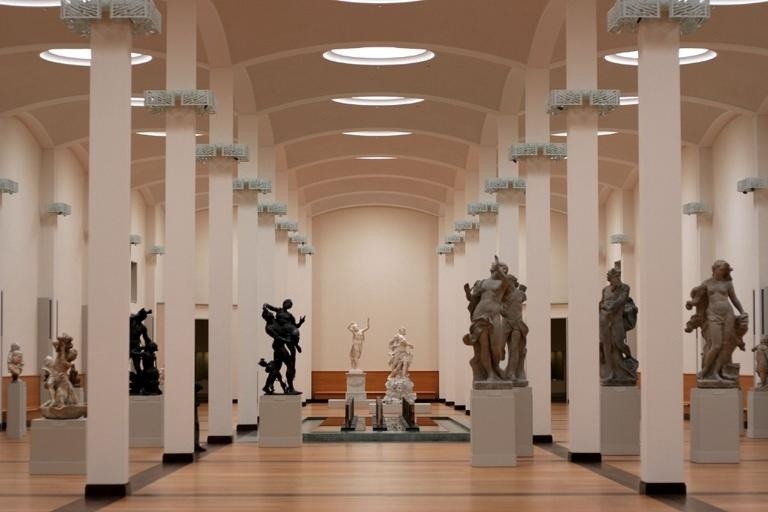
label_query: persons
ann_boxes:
[258,299,306,395]
[684,259,749,382]
[752,334,768,387]
[129,307,160,395]
[40,332,80,408]
[598,268,639,385]
[385,326,415,378]
[7,342,24,383]
[463,254,529,382]
[345,318,370,369]
